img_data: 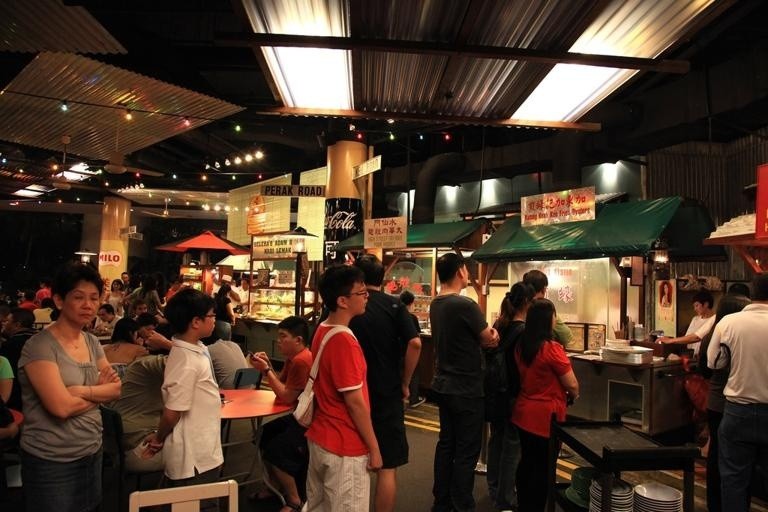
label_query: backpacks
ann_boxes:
[487,319,526,399]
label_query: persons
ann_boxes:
[655,272,768,512]
[0,253,580,512]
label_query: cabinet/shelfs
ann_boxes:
[542,411,703,512]
[179,263,232,296]
[249,230,319,321]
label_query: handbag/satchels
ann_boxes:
[293,390,314,428]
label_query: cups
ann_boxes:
[635,327,645,342]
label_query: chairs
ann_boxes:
[0,305,300,511]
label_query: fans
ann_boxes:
[17,135,105,193]
[59,104,166,179]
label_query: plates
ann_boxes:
[565,467,684,512]
[266,316,283,322]
[601,345,653,355]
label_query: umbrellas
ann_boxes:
[153,228,250,265]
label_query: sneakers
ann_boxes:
[410,395,426,407]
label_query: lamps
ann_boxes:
[73,248,99,266]
[648,240,677,275]
[280,224,318,320]
